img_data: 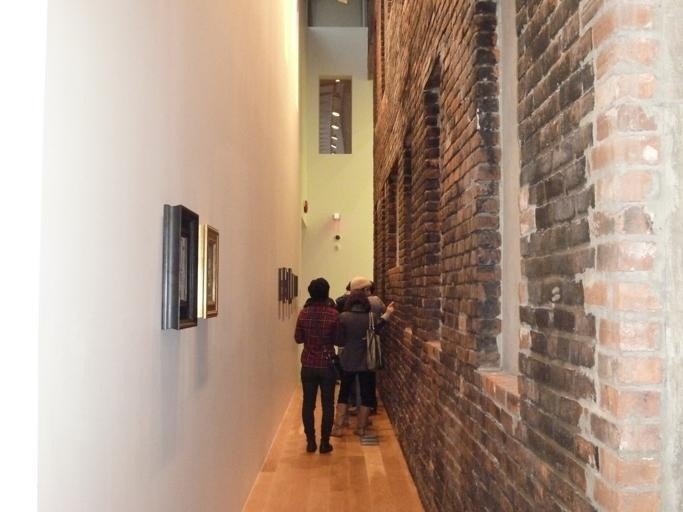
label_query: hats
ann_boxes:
[350,277,372,291]
[308,278,329,296]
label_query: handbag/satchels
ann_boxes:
[331,355,342,380]
[366,329,384,373]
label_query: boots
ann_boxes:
[353,406,370,436]
[320,438,332,453]
[307,436,317,451]
[331,403,348,437]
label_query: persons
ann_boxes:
[295,278,346,454]
[330,278,395,437]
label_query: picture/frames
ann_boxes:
[162,202,218,331]
